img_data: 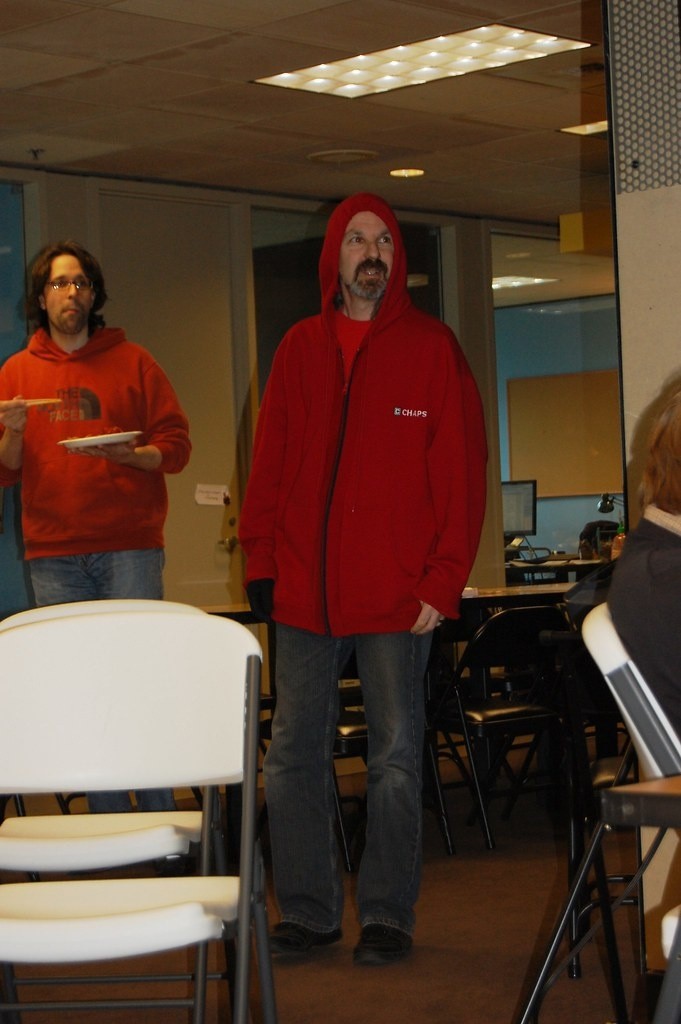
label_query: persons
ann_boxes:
[233,196,496,975]
[0,242,192,879]
[607,375,681,815]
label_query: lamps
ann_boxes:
[596,493,623,513]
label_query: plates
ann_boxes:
[57,431,143,448]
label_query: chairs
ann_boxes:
[578,520,622,582]
[1,599,278,1024]
[513,603,681,1024]
[0,601,608,872]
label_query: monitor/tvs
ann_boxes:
[500,480,537,536]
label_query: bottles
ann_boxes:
[611,527,626,561]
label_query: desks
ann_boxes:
[509,553,603,583]
[197,582,580,626]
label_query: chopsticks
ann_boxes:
[21,399,63,406]
